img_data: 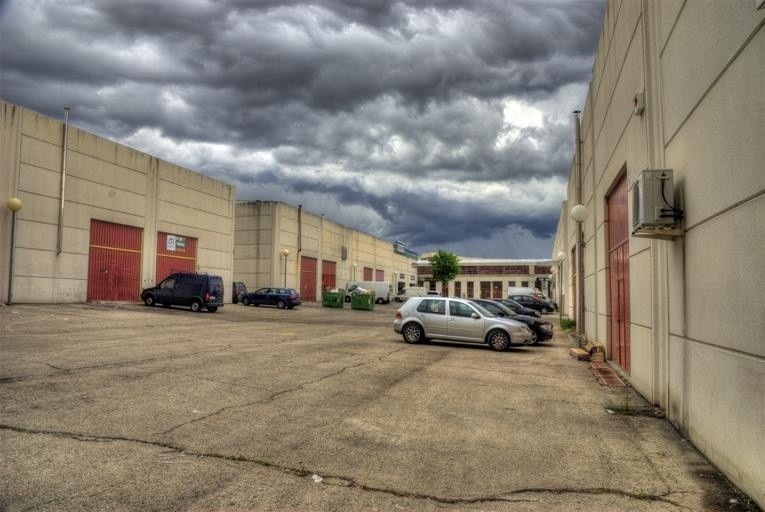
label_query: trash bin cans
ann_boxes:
[352,292,375,310]
[322,292,345,308]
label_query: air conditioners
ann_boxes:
[633,169,674,233]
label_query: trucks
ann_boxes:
[507,286,546,298]
[345,281,394,306]
[394,286,428,302]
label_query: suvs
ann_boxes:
[140,272,225,313]
[241,288,301,309]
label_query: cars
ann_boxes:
[392,297,537,350]
[497,299,541,317]
[464,298,554,342]
[427,291,441,297]
[506,295,554,313]
[232,281,248,305]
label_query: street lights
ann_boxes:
[548,266,557,306]
[281,248,291,289]
[351,262,358,282]
[557,251,567,327]
[4,196,23,304]
[568,203,591,352]
[393,271,398,297]
